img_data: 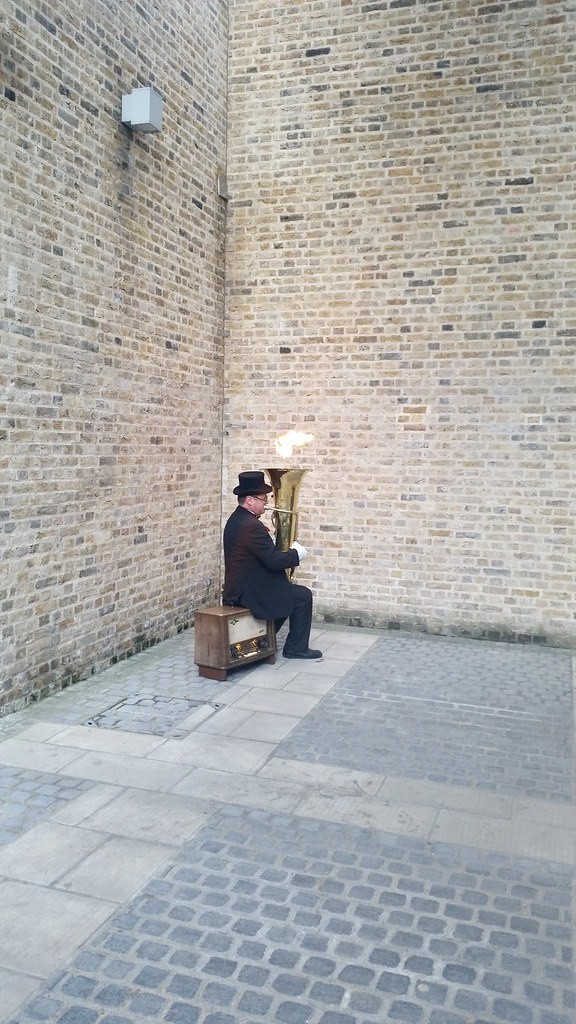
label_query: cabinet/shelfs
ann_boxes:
[193,604,277,681]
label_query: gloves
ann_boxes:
[290,541,307,561]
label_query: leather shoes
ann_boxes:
[258,639,268,648]
[282,643,322,658]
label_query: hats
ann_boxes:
[233,471,272,495]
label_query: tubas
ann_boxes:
[258,468,314,583]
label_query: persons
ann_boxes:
[223,471,322,659]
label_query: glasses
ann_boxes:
[252,496,267,502]
